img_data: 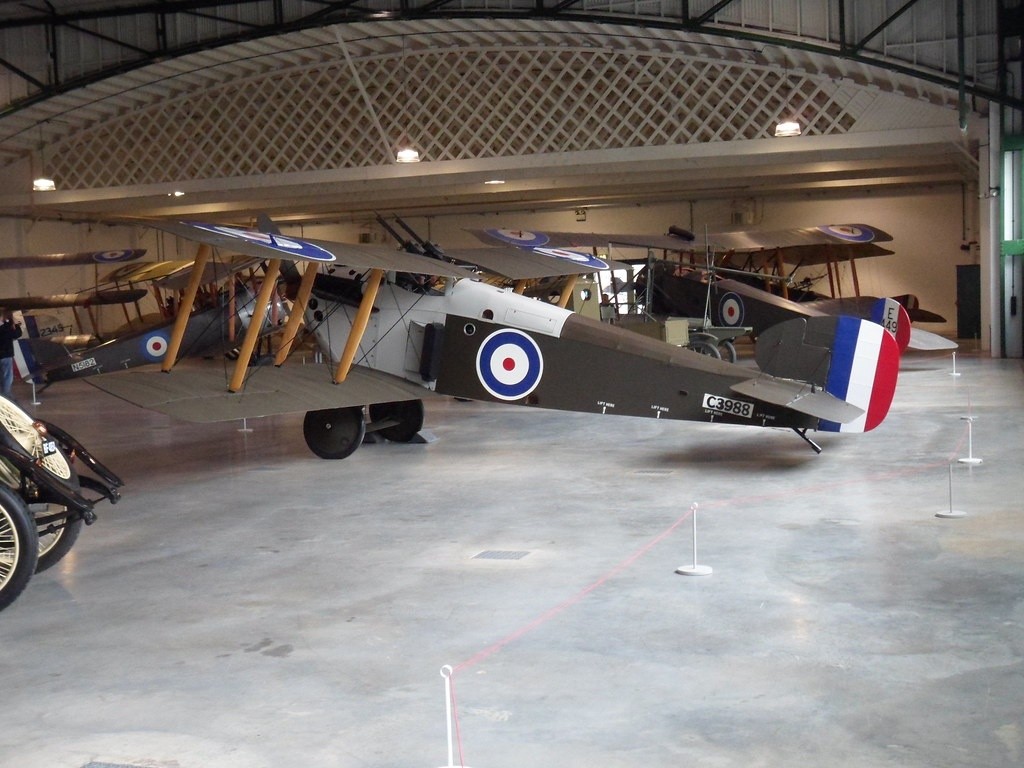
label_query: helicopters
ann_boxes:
[132,213,901,461]
[0,214,454,398]
[473,221,959,368]
